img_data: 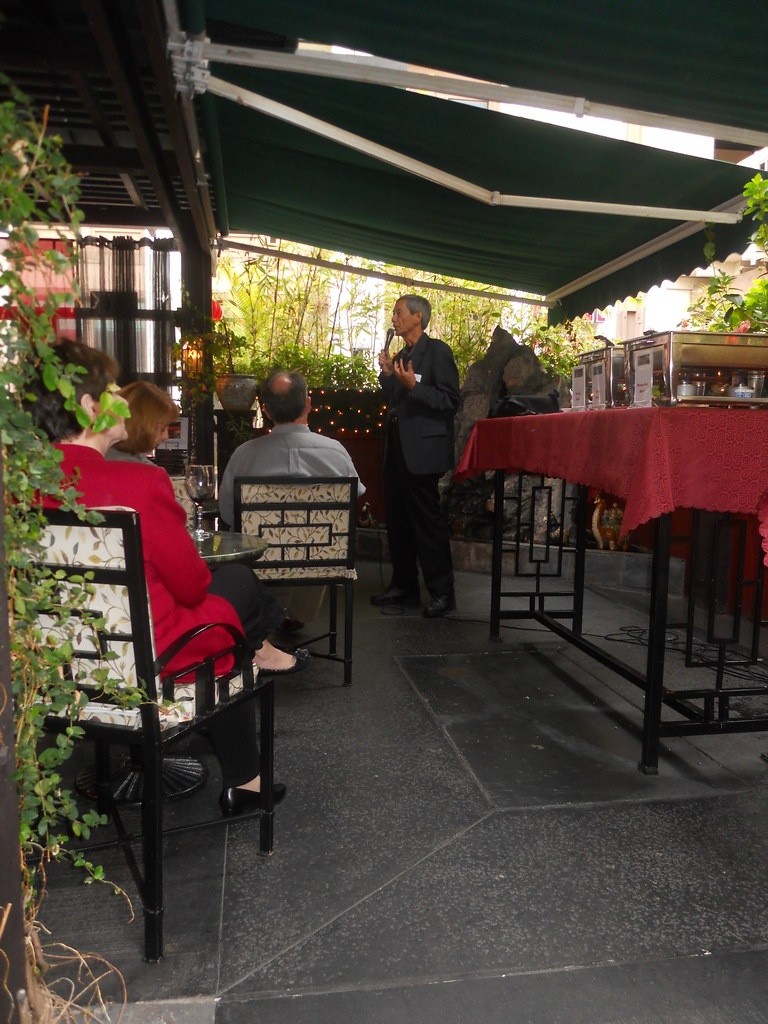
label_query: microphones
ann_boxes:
[384,328,394,351]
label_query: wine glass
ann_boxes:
[184,464,215,542]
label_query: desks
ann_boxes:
[72,528,267,807]
[454,407,768,775]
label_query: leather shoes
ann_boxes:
[219,783,287,819]
[370,585,420,608]
[258,649,314,679]
[424,596,456,618]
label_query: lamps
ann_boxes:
[183,336,205,465]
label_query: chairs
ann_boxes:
[233,475,359,687]
[18,506,278,965]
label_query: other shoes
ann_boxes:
[276,619,304,633]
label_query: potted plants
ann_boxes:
[170,317,259,410]
[251,344,389,437]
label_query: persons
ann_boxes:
[218,370,368,639]
[23,339,315,824]
[371,294,464,619]
[106,381,182,479]
